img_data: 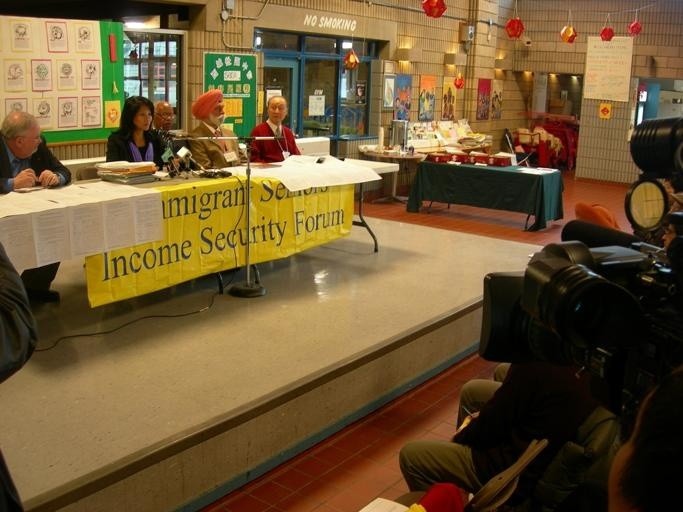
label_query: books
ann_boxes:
[13,186,46,193]
[94,158,159,187]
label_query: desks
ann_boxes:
[369,141,561,231]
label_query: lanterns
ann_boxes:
[422,1,447,21]
[343,39,360,72]
[597,12,614,43]
[505,0,524,44]
[559,9,577,46]
[626,8,644,38]
[453,74,466,91]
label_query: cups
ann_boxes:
[378,127,384,154]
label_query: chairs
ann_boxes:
[505,128,535,168]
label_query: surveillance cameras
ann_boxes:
[520,35,532,46]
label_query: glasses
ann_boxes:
[156,113,175,120]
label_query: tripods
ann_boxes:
[178,158,201,179]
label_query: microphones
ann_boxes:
[159,146,181,176]
[157,129,176,137]
[172,144,207,171]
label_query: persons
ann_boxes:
[153,100,187,171]
[0,112,72,308]
[399,176,683,512]
[105,96,167,175]
[247,95,302,164]
[182,89,249,169]
[394,86,411,119]
[0,243,38,511]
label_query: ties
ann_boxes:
[215,132,224,144]
[276,128,281,138]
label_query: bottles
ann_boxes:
[409,146,414,156]
